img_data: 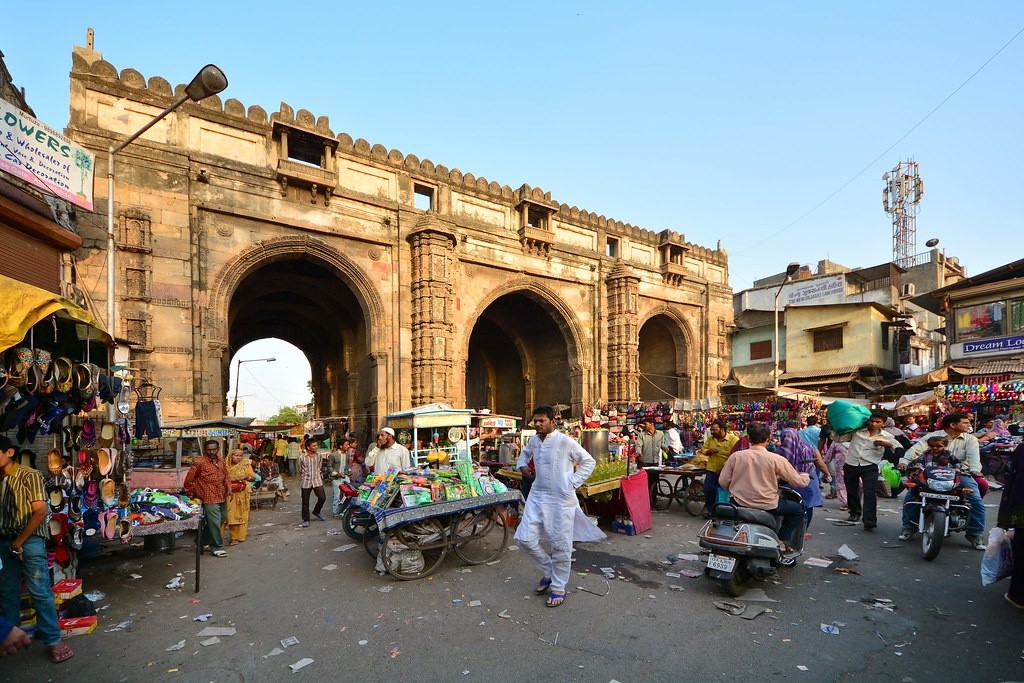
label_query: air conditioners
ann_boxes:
[903,283,915,296]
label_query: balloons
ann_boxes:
[944,382,1024,401]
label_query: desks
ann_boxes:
[70,504,206,594]
[249,492,279,511]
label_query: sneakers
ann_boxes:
[898,530,913,541]
[965,534,986,550]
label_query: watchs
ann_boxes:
[9,548,24,555]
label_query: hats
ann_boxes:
[381,427,395,437]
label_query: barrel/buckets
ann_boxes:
[144,533,171,549]
[582,430,609,463]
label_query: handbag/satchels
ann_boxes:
[981,527,1014,587]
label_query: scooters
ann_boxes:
[694,456,819,598]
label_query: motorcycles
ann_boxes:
[890,465,980,561]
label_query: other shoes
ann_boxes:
[0,347,134,569]
[847,515,860,522]
[303,521,310,527]
[230,541,238,545]
[313,514,325,521]
[864,521,877,529]
[1005,593,1024,608]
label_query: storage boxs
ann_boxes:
[611,520,635,536]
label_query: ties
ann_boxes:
[0,476,8,531]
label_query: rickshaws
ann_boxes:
[341,402,526,582]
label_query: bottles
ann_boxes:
[585,453,628,485]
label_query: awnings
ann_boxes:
[802,320,849,333]
[721,357,1024,394]
[881,320,945,336]
[0,271,120,356]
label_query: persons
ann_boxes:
[997,438,1024,608]
[718,424,811,554]
[329,437,363,518]
[0,435,74,663]
[700,420,761,518]
[453,431,485,465]
[516,406,597,608]
[897,413,989,551]
[365,427,411,476]
[219,448,256,545]
[772,427,832,540]
[183,440,231,557]
[296,437,327,527]
[242,432,311,502]
[900,412,1010,440]
[635,418,668,509]
[796,411,913,530]
[664,422,695,461]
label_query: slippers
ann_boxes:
[534,579,551,594]
[194,544,211,551]
[703,513,712,517]
[840,505,848,510]
[780,544,794,554]
[213,551,228,557]
[826,494,837,498]
[51,645,74,662]
[547,593,566,607]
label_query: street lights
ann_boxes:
[233,357,277,417]
[771,261,802,397]
[101,62,230,448]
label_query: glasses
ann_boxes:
[928,444,945,447]
